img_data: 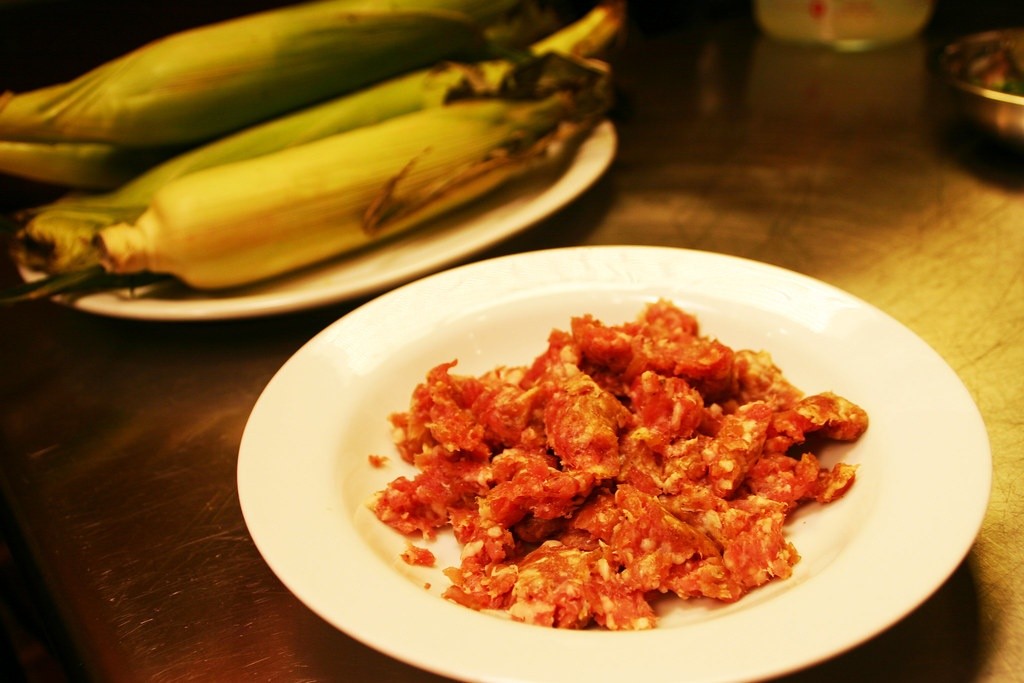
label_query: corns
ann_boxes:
[0,1,623,293]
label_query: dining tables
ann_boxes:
[1,0,1024,683]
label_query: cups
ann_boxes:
[751,0,939,51]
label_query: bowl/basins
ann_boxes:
[930,29,1024,155]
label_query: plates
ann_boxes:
[16,115,619,320]
[237,246,996,681]
